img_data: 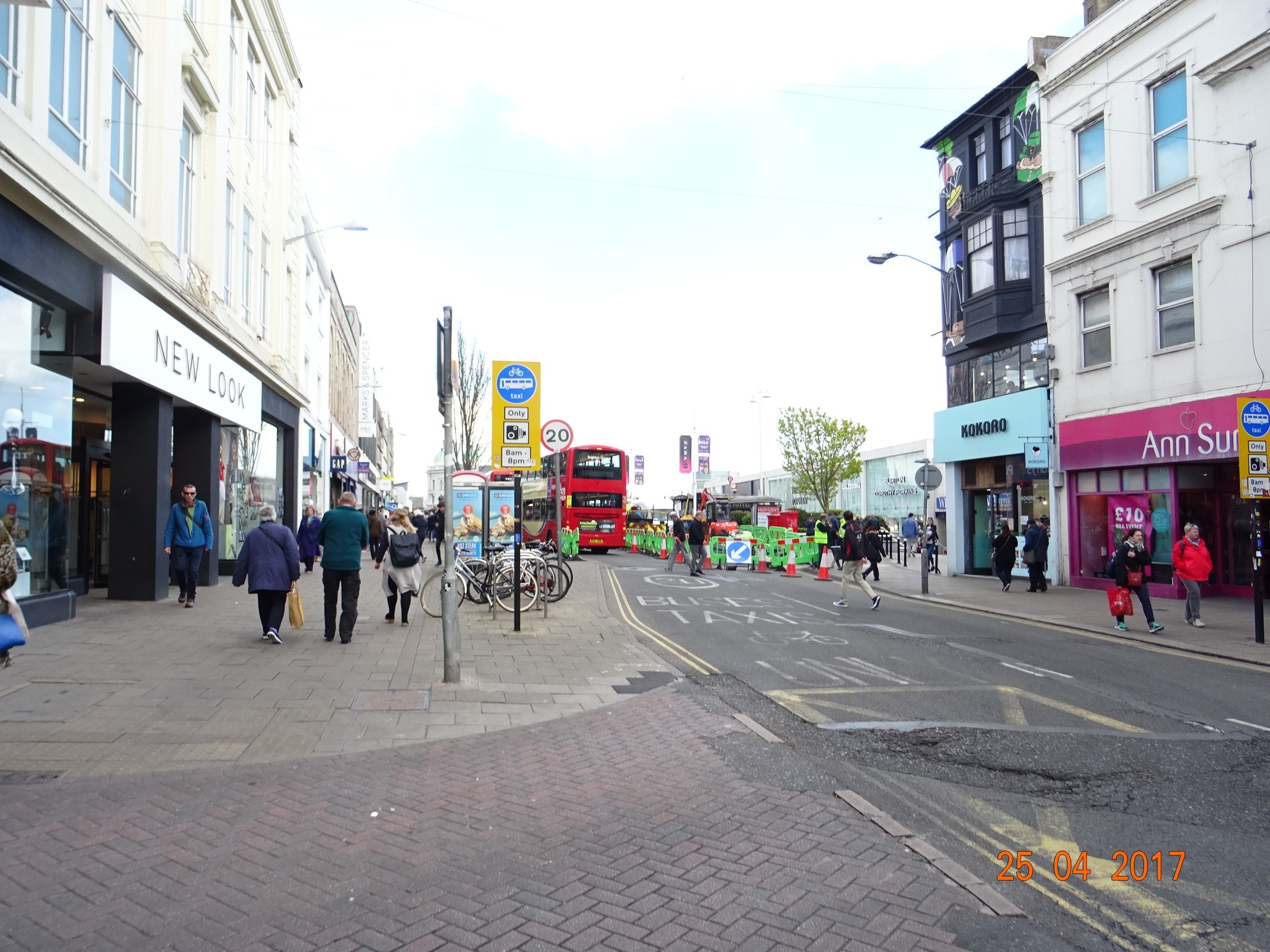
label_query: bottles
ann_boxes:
[3,504,19,544]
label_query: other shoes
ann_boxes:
[1001,582,1048,593]
[907,551,935,572]
[696,569,705,575]
[341,638,351,644]
[262,632,269,639]
[862,573,880,581]
[662,567,673,572]
[1185,618,1205,627]
[0,652,12,670]
[385,612,394,623]
[402,618,408,626]
[690,572,699,577]
[325,635,334,641]
[267,627,282,644]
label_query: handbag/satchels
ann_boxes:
[990,549,1035,566]
[1106,587,1134,616]
[1127,571,1142,587]
[1172,540,1183,571]
[872,534,882,562]
[287,580,304,629]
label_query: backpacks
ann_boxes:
[385,526,420,568]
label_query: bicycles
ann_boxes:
[420,538,574,618]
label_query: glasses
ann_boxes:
[184,492,196,495]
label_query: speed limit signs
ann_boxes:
[541,420,573,453]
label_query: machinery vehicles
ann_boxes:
[703,500,739,554]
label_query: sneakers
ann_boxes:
[833,600,847,607]
[1114,621,1129,631]
[185,598,194,607]
[870,596,881,610]
[178,591,186,603]
[1148,622,1164,633]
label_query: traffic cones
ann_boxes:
[674,550,685,564]
[813,545,833,581]
[702,538,717,570]
[780,543,802,578]
[753,543,772,574]
[628,531,639,553]
[658,534,668,559]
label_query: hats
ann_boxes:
[809,513,814,516]
[1026,519,1036,528]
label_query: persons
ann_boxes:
[992,525,1018,591]
[688,510,708,577]
[861,526,886,581]
[232,504,301,643]
[1034,514,1051,590]
[833,510,881,609]
[318,491,369,644]
[356,502,446,566]
[296,505,322,573]
[0,515,18,671]
[662,512,697,573]
[924,517,939,573]
[163,484,214,608]
[1171,523,1213,627]
[1021,519,1049,592]
[814,513,832,569]
[805,512,880,569]
[901,513,924,557]
[374,508,421,627]
[1114,528,1164,633]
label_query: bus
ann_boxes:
[0,439,72,485]
[486,445,628,554]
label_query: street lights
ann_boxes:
[749,390,771,497]
[913,458,931,594]
